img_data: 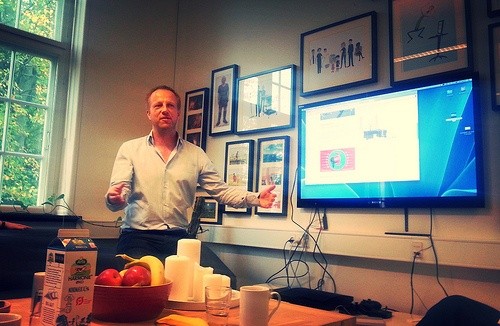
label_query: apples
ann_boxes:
[123,265,150,287]
[95,268,122,286]
[119,269,128,277]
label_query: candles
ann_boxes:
[165,253,190,298]
[178,238,201,296]
[193,262,213,299]
[206,273,230,299]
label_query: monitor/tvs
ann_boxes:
[297,70,486,208]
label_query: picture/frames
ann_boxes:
[196,196,221,225]
[226,140,254,213]
[254,136,290,215]
[209,63,237,136]
[182,87,210,151]
[388,0,472,85]
[235,63,296,135]
[300,10,378,95]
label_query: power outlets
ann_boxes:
[411,242,423,257]
[290,232,307,246]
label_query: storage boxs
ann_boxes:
[40,228,98,326]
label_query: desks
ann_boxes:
[8,298,357,326]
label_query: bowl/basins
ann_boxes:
[0,313,21,326]
[89,280,173,323]
[0,300,11,313]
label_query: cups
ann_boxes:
[205,284,230,326]
[238,286,281,326]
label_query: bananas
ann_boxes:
[115,254,164,286]
[154,314,209,326]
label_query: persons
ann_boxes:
[104,85,277,272]
[216,76,229,126]
